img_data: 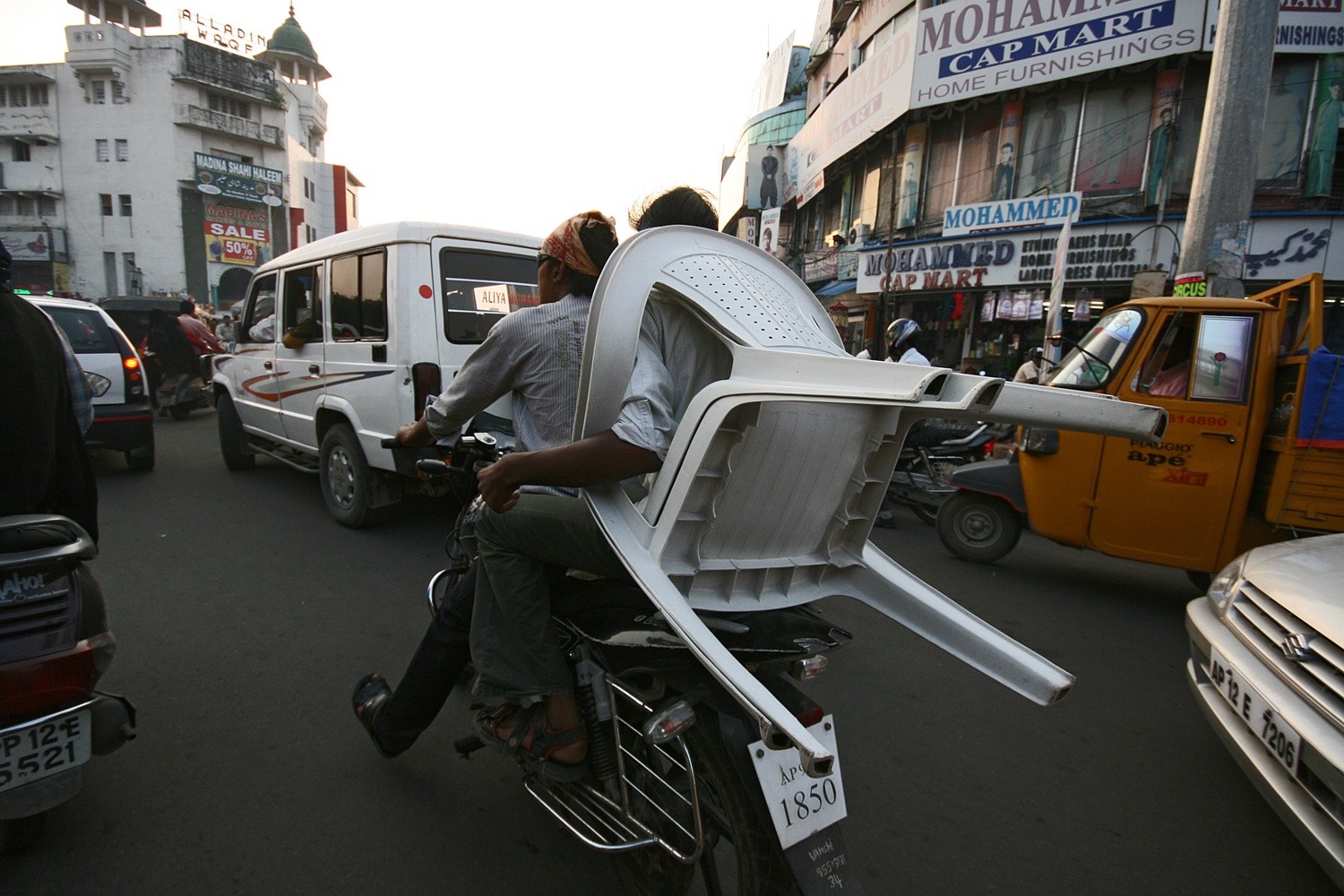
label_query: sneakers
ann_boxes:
[872,516,897,530]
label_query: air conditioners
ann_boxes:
[847,223,871,245]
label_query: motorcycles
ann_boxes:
[936,271,1344,591]
[888,415,1002,528]
[380,361,857,896]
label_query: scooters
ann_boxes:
[153,338,230,421]
[0,512,137,856]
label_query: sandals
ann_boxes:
[472,701,595,785]
[351,672,404,759]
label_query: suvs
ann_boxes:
[205,219,545,530]
[96,292,196,390]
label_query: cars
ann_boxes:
[1185,532,1344,896]
[17,293,156,474]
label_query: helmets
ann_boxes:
[885,318,923,363]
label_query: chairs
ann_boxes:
[570,224,1169,776]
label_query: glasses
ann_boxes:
[536,251,553,270]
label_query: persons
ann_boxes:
[249,278,332,349]
[163,299,237,390]
[1154,316,1221,400]
[351,188,749,779]
[1255,63,1305,185]
[760,145,778,208]
[855,345,875,360]
[901,162,916,226]
[761,228,773,256]
[211,233,225,261]
[253,240,271,267]
[1148,108,1176,204]
[1090,95,1124,185]
[995,142,1014,202]
[1010,351,1044,386]
[1305,78,1344,196]
[1030,95,1066,193]
[881,318,934,367]
